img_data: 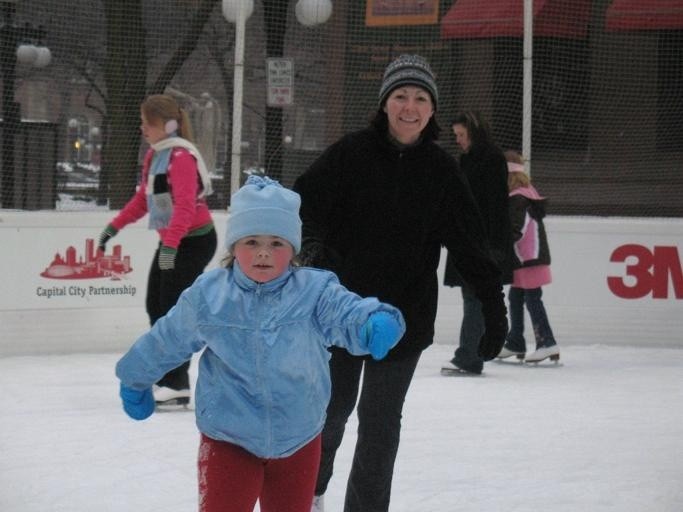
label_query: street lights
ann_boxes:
[220,0,333,183]
[0,21,53,209]
[67,119,82,157]
[87,121,99,162]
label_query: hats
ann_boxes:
[376,53,438,112]
[224,175,303,257]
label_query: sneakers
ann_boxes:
[525,344,559,362]
[151,386,189,404]
[496,346,524,358]
[310,493,324,512]
[442,361,468,373]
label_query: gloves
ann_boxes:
[97,228,111,252]
[302,241,340,270]
[357,310,400,360]
[119,380,154,420]
[157,245,177,273]
[478,305,507,362]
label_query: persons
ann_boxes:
[99,94,217,404]
[501,150,561,363]
[290,53,508,512]
[440,109,514,377]
[115,174,407,511]
